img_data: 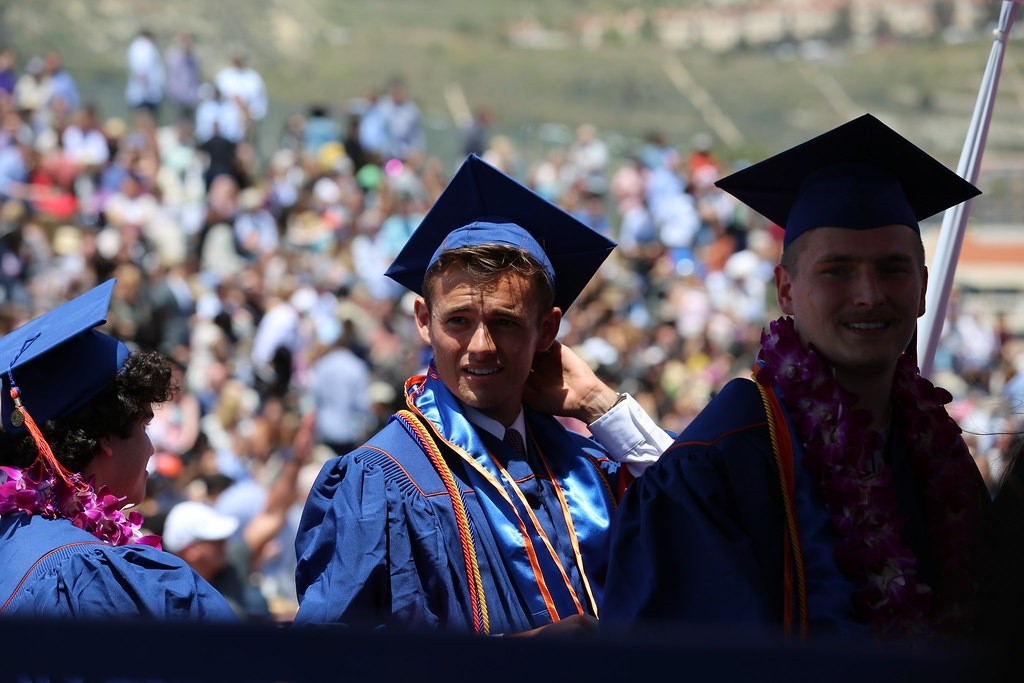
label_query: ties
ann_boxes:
[502,429,542,512]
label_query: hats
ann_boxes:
[162,501,239,554]
[0,277,132,434]
[383,154,619,318]
[714,113,983,249]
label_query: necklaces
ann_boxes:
[2,466,163,550]
[752,315,982,637]
[389,403,619,637]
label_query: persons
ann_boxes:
[599,113,997,640]
[1,276,242,624]
[1,26,1024,618]
[295,153,681,638]
